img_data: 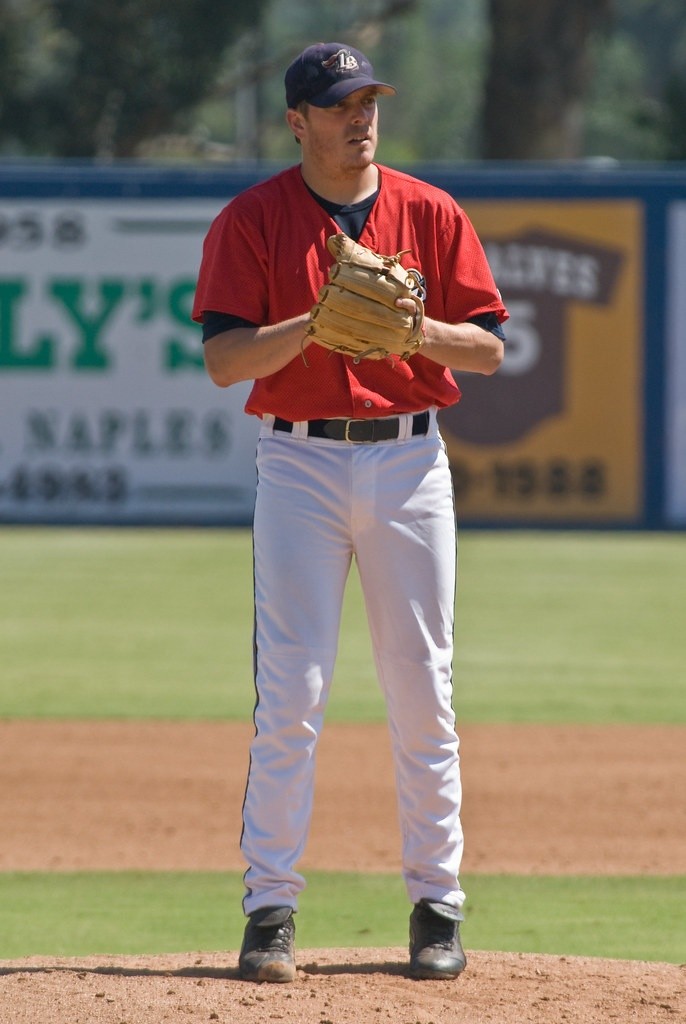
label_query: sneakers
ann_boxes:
[238,905,295,982]
[408,900,467,978]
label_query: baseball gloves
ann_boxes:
[297,233,427,371]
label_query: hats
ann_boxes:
[285,42,398,109]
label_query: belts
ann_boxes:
[271,411,430,443]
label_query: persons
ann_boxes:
[191,41,510,980]
